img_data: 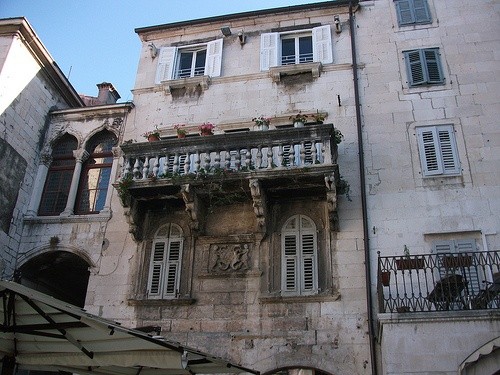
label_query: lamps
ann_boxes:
[220,26,232,36]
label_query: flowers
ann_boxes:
[252,113,272,126]
[198,121,216,132]
[140,126,161,139]
[172,123,192,134]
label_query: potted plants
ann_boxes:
[395,244,423,270]
[289,110,308,127]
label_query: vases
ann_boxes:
[148,135,159,142]
[258,124,268,130]
[202,131,209,136]
[178,133,184,138]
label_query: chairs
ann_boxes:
[425,272,500,309]
